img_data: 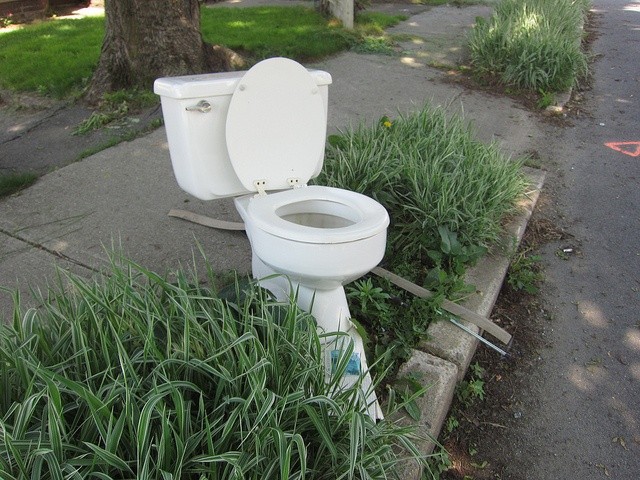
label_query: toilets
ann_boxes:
[151,55,390,422]
